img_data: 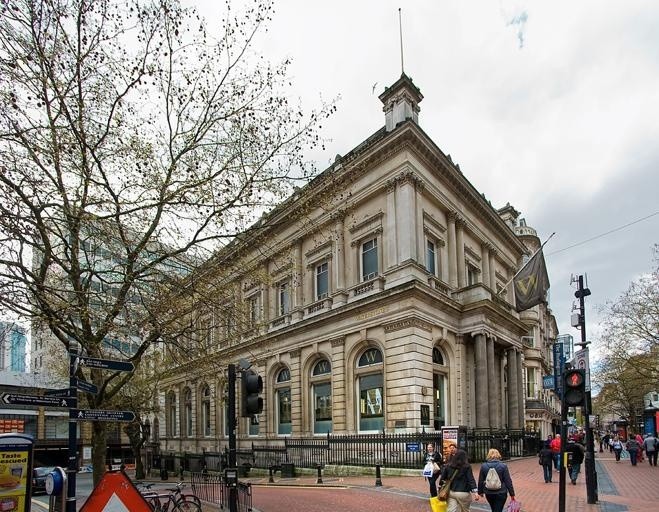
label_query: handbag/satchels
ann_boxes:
[508,500,522,512]
[423,461,440,478]
[537,456,544,465]
[429,480,451,512]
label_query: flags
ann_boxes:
[514,250,551,313]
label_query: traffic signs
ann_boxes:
[70,377,98,393]
[68,409,136,423]
[71,355,136,373]
[43,388,78,398]
[2,393,76,408]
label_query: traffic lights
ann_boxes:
[563,368,586,407]
[242,369,263,418]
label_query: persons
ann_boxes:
[438,450,479,512]
[422,443,442,497]
[593,429,659,466]
[445,444,457,462]
[477,448,515,511]
[538,429,585,485]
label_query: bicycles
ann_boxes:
[133,480,202,512]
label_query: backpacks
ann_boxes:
[484,461,502,491]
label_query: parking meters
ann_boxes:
[46,467,66,512]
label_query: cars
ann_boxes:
[32,466,56,494]
